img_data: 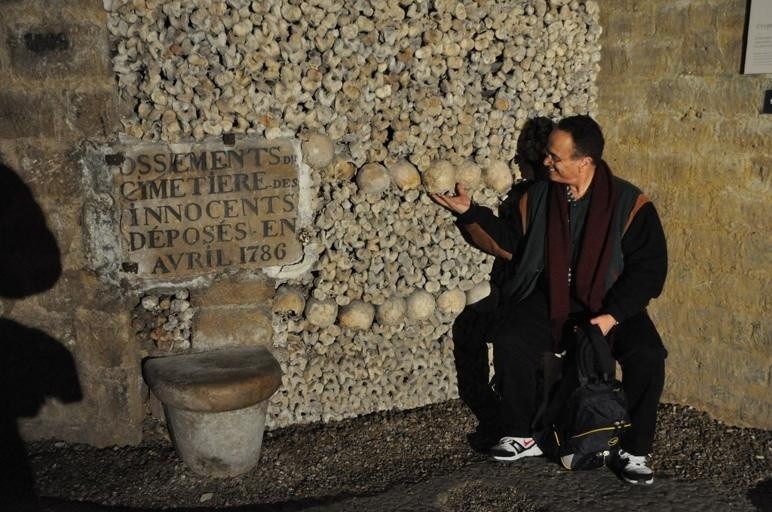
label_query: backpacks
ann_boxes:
[545,325,633,470]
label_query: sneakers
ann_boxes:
[615,450,655,486]
[489,436,544,462]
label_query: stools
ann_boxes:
[143,344,283,478]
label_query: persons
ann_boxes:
[427,116,668,485]
